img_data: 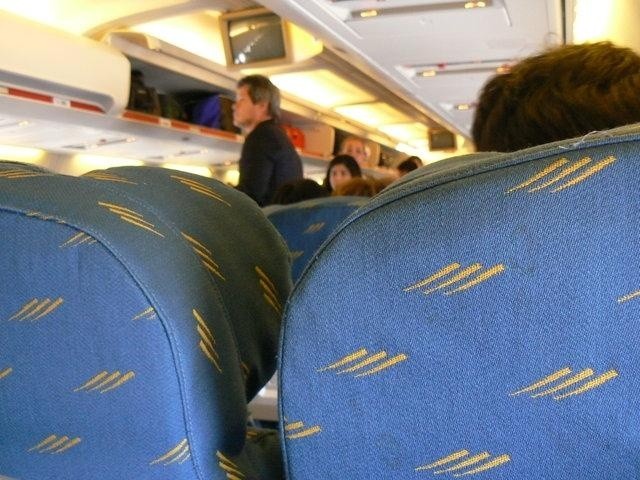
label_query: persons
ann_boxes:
[471,39,640,153]
[222,75,425,208]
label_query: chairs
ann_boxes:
[262,196,377,284]
[80,165,292,402]
[0,159,248,479]
[276,122,639,480]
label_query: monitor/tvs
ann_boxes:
[220,6,292,71]
[428,128,457,152]
